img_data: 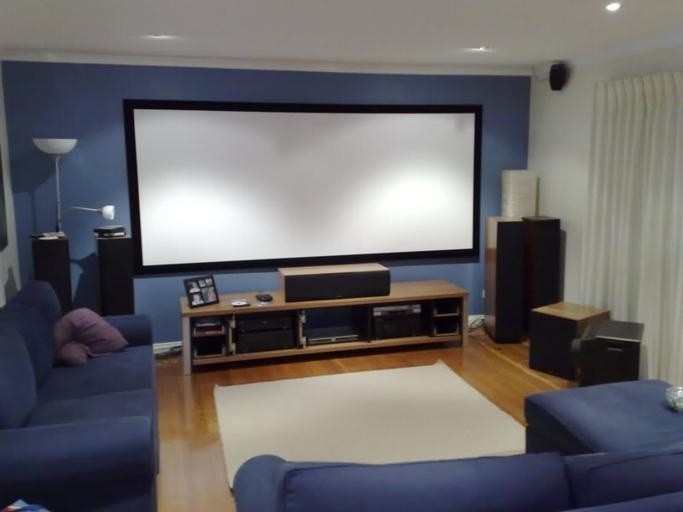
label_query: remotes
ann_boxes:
[256,295,272,302]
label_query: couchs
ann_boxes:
[232,376,683,510]
[1,278,156,512]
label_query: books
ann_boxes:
[93,230,126,237]
[195,318,221,331]
[93,224,124,232]
[596,320,645,343]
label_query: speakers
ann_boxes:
[96,234,134,317]
[276,267,391,303]
[485,217,526,343]
[550,64,566,90]
[523,216,561,335]
[594,321,641,387]
[33,238,71,315]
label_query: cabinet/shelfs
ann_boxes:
[180,280,474,373]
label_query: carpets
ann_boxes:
[212,355,529,487]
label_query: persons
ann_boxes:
[187,278,217,306]
